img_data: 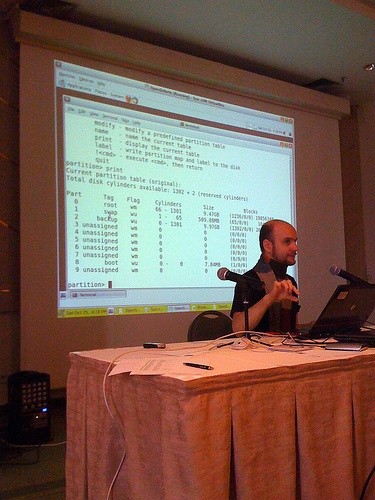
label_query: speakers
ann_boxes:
[7,370,51,445]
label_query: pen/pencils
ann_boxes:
[181,359,216,372]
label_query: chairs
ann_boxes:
[188,310,232,342]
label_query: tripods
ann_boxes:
[217,288,274,348]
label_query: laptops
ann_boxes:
[268,284,375,338]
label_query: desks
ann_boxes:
[66,335,375,500]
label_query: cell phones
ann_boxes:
[143,343,165,349]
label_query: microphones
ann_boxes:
[217,267,266,290]
[329,266,370,285]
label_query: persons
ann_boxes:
[229,220,301,336]
